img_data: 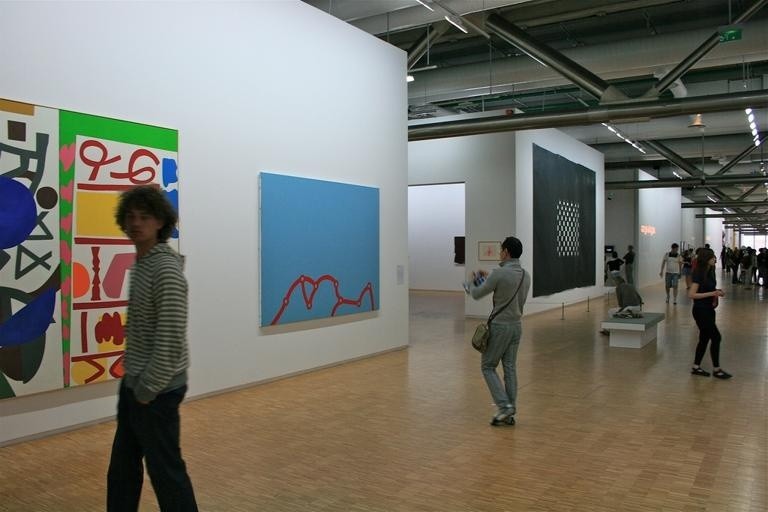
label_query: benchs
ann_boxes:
[601,309,666,350]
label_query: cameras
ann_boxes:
[474,271,484,286]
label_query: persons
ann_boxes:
[720,243,768,289]
[600,275,644,335]
[687,248,732,379]
[659,242,684,304]
[464,234,532,426]
[621,245,636,286]
[607,252,624,285]
[604,253,608,283]
[104,183,203,512]
[678,244,712,290]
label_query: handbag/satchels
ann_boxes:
[713,290,718,309]
[471,324,490,353]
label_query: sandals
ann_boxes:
[691,366,732,379]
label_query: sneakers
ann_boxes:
[491,405,517,426]
[600,329,609,336]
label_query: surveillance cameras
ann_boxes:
[608,193,613,200]
